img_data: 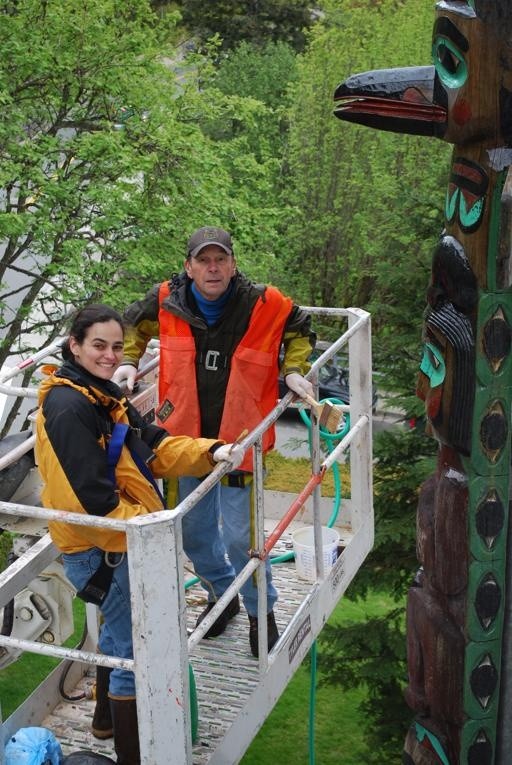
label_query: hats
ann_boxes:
[186,226,233,257]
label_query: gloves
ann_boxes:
[110,364,139,392]
[285,371,316,406]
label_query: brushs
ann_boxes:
[300,393,342,433]
[229,429,249,455]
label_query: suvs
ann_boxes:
[273,347,379,422]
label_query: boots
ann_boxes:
[107,690,142,764]
[89,644,114,740]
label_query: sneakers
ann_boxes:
[246,609,279,659]
[195,591,241,638]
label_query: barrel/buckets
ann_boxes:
[291,526,340,582]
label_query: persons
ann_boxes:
[35,301,247,765]
[108,226,316,661]
